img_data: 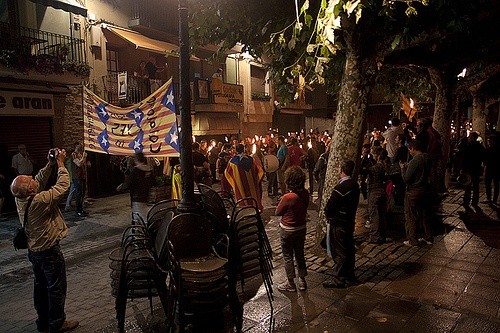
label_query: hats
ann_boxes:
[9,175,33,198]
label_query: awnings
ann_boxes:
[27,0,88,17]
[99,22,202,63]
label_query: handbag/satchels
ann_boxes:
[320,227,327,249]
[11,194,37,249]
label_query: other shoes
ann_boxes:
[403,241,419,248]
[34,320,79,333]
[75,210,89,217]
[418,238,432,245]
[323,278,356,288]
[463,198,497,209]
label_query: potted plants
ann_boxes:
[130,174,173,225]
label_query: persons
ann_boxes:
[12,143,36,178]
[10,148,79,333]
[132,70,139,77]
[213,68,224,80]
[118,116,500,227]
[274,164,309,292]
[64,139,91,218]
[361,144,392,245]
[395,136,435,247]
[139,60,151,80]
[322,160,356,288]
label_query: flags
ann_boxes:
[83,76,181,157]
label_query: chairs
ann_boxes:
[108,184,275,333]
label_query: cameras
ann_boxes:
[49,150,61,161]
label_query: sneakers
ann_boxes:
[277,282,296,292]
[298,281,307,291]
